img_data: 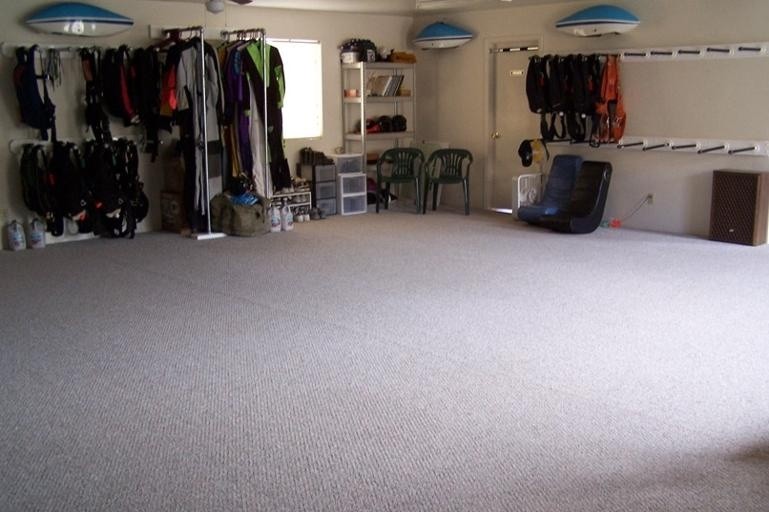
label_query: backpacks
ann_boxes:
[13,45,50,130]
[526,54,601,115]
[20,143,118,216]
[104,45,136,118]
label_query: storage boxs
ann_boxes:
[297,152,367,216]
[161,192,188,232]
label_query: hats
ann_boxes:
[518,140,531,167]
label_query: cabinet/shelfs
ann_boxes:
[269,192,312,211]
[341,61,417,209]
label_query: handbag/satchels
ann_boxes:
[222,190,271,236]
[210,193,222,232]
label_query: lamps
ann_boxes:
[554,5,641,37]
[206,0,224,14]
[24,1,134,38]
[411,21,473,51]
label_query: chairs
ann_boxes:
[518,155,582,226]
[376,147,425,214]
[423,148,473,216]
[538,161,612,234]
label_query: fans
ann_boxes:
[230,0,253,6]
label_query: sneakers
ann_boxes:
[302,147,333,165]
[272,176,325,222]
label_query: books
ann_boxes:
[373,75,403,96]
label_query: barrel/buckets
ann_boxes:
[281,198,294,231]
[268,207,281,232]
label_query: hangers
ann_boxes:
[220,27,263,52]
[152,25,200,52]
